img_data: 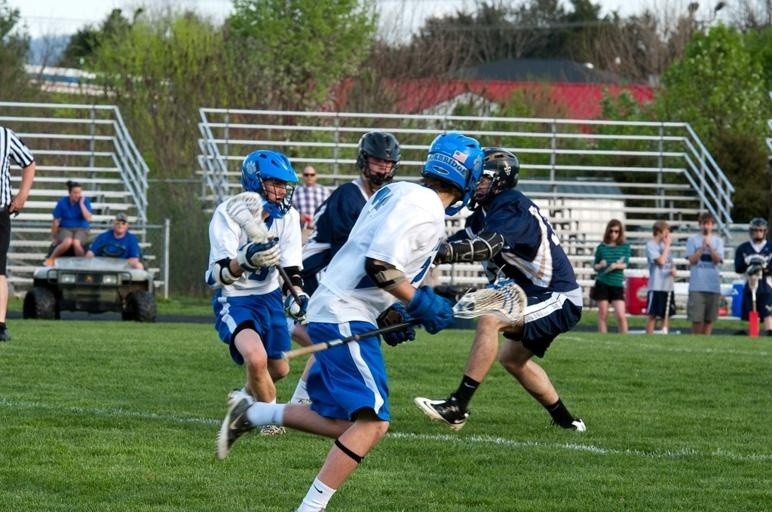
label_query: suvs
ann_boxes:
[24,254,158,324]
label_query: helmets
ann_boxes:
[241,149,300,211]
[469,147,521,209]
[355,130,403,191]
[422,133,486,218]
[747,218,769,243]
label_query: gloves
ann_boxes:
[746,255,768,267]
[283,291,312,326]
[404,283,455,336]
[431,244,453,266]
[376,301,417,347]
[236,235,282,270]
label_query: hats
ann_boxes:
[113,212,129,223]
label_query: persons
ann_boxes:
[589,219,631,334]
[292,165,332,247]
[84,212,144,270]
[0,125,35,342]
[216,132,486,512]
[645,221,681,335]
[413,147,588,433]
[288,132,402,405]
[41,181,93,267]
[204,149,311,437]
[736,217,772,337]
[684,213,724,335]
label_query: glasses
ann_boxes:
[609,229,620,234]
[302,172,318,178]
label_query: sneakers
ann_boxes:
[0,321,12,342]
[214,386,258,463]
[287,398,313,407]
[262,423,287,437]
[414,395,471,432]
[550,418,587,434]
